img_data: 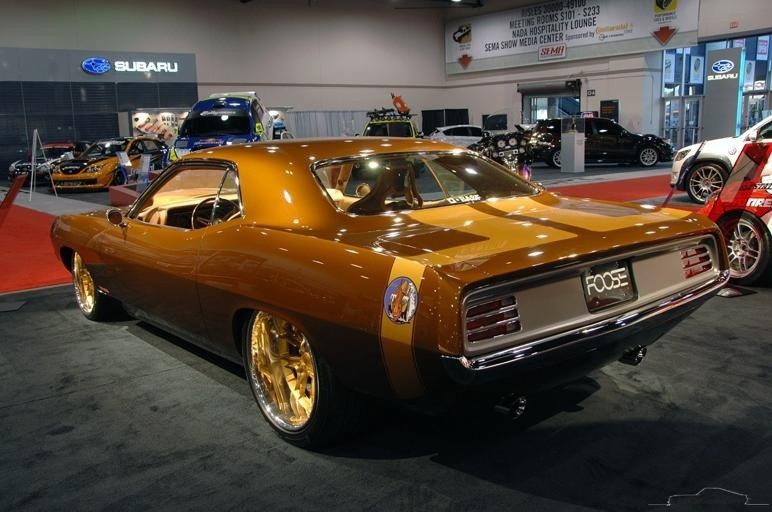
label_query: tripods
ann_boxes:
[30,129,58,201]
